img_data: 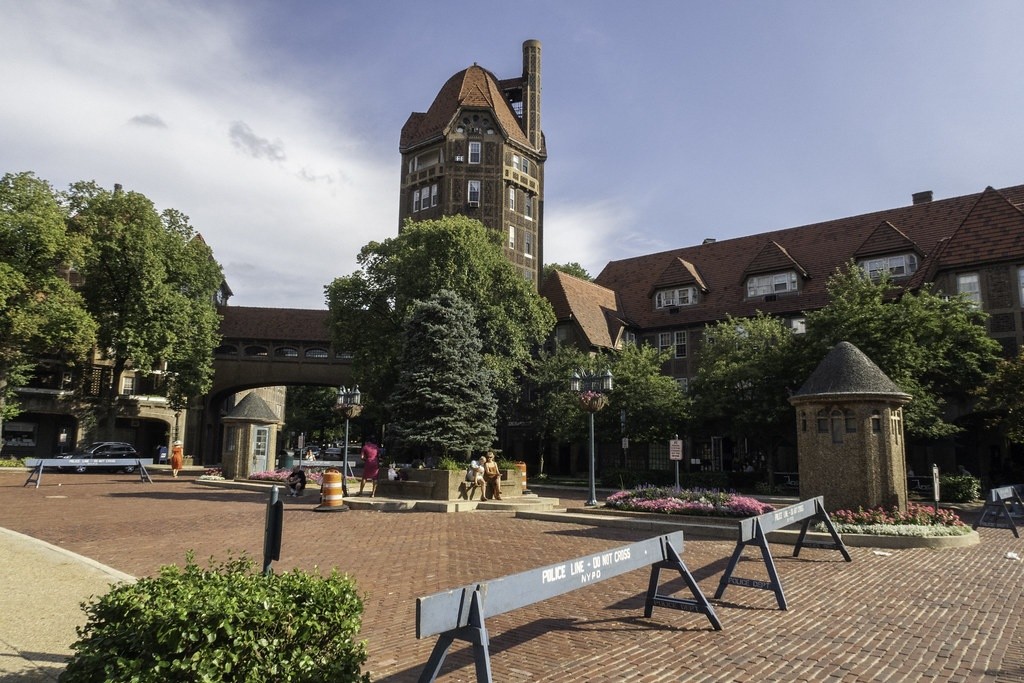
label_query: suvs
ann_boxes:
[51,441,141,474]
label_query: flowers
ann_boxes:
[580,391,608,407]
[606,483,775,517]
[199,470,225,481]
[249,467,325,483]
[814,503,973,538]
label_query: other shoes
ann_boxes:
[495,495,502,500]
[481,496,488,500]
[370,494,374,497]
[474,483,479,488]
[496,490,502,493]
[355,493,363,497]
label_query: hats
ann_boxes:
[173,440,183,446]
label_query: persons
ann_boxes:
[485,451,504,500]
[283,464,307,498]
[466,456,489,502]
[356,438,382,498]
[304,449,316,461]
[171,440,183,477]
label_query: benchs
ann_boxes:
[461,480,516,500]
[375,479,436,499]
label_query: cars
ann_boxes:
[302,446,322,458]
[322,447,344,461]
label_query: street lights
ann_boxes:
[570,365,614,506]
[335,382,361,498]
